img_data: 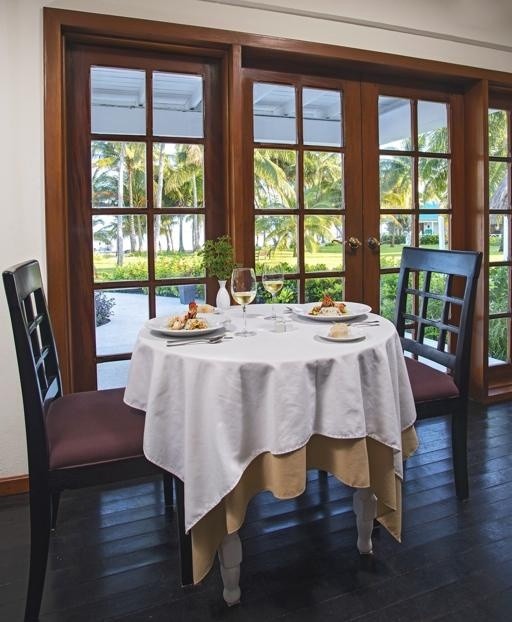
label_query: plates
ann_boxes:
[318,331,365,341]
[148,314,224,337]
[181,305,221,314]
[290,301,372,321]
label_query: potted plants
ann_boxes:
[196,233,238,319]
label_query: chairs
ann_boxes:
[2,258,199,622]
[309,242,486,531]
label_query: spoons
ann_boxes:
[167,335,225,347]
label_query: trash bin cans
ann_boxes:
[178,284,197,305]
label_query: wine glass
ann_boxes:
[262,267,285,320]
[231,267,257,335]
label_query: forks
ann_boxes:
[331,320,379,326]
